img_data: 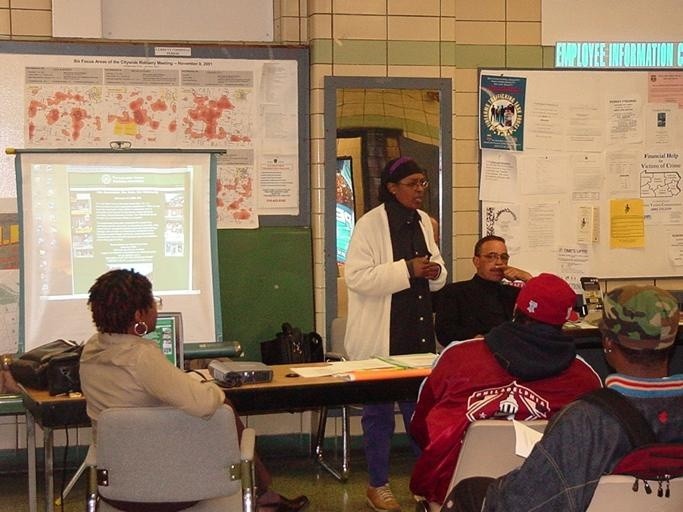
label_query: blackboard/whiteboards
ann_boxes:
[477,66,683,282]
[0,39,312,229]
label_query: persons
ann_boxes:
[439,286,683,512]
[79,269,309,512]
[434,235,534,347]
[343,157,448,512]
[409,273,605,506]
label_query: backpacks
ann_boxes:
[578,388,683,497]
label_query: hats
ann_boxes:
[516,273,576,325]
[584,284,680,349]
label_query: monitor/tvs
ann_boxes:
[142,312,184,372]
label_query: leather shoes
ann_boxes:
[257,494,308,512]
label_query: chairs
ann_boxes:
[87,404,257,512]
[585,474,682,512]
[413,419,552,512]
[314,317,403,484]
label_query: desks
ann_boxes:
[18,351,437,512]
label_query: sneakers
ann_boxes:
[367,485,400,512]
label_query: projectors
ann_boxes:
[208,359,273,387]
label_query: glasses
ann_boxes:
[481,253,510,262]
[399,181,428,189]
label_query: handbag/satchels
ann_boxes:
[10,340,83,396]
